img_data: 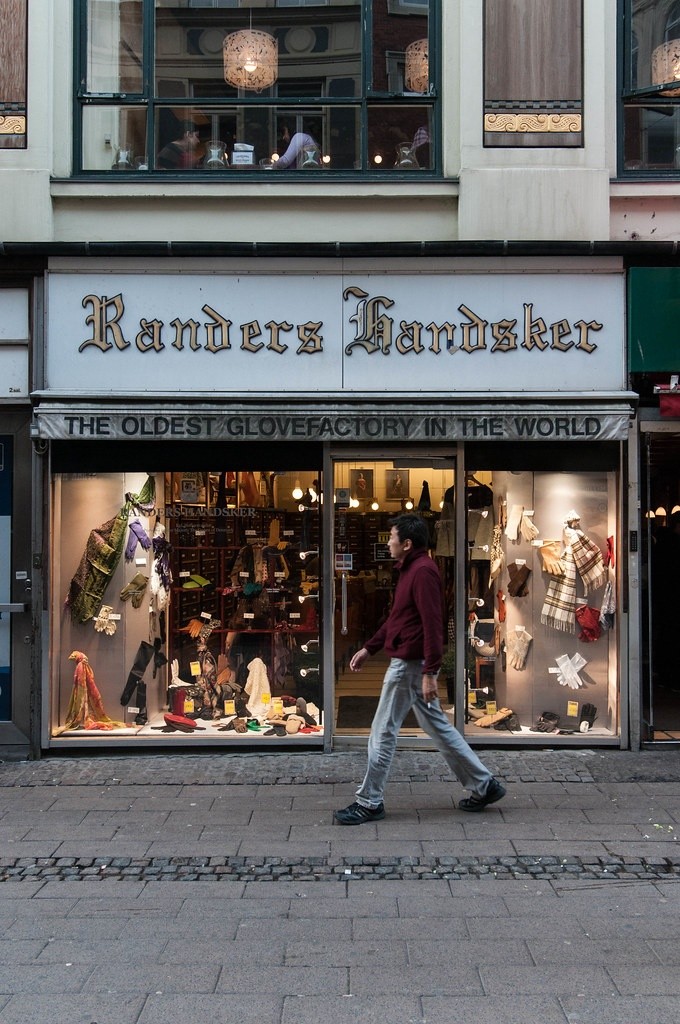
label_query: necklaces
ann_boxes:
[332,514,506,825]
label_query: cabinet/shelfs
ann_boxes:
[165,504,441,685]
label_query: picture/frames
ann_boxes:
[384,468,411,500]
[349,468,375,500]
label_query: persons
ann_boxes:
[272,118,322,171]
[223,541,291,662]
[155,119,205,171]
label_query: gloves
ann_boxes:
[504,504,541,542]
[496,590,505,622]
[474,707,514,727]
[507,561,532,598]
[494,712,522,731]
[179,617,203,637]
[151,610,168,678]
[125,521,151,562]
[539,540,565,575]
[561,703,597,733]
[575,606,600,646]
[606,536,614,569]
[160,652,322,737]
[267,519,292,551]
[507,628,531,672]
[529,712,560,733]
[556,652,588,690]
[121,642,155,724]
[500,640,506,672]
[488,624,501,647]
[182,573,211,589]
[118,574,148,610]
[92,606,117,637]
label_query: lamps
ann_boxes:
[366,497,379,512]
[401,497,415,512]
[405,38,429,92]
[651,38,680,97]
[223,8,279,91]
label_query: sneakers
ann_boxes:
[336,802,387,825]
[459,780,506,811]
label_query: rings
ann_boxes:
[432,695,436,699]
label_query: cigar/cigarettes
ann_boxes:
[427,701,431,709]
